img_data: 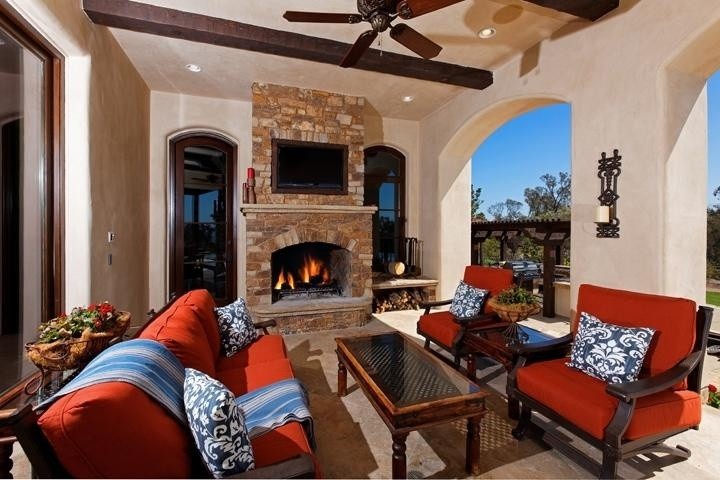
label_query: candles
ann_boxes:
[248,168,255,178]
[595,205,609,222]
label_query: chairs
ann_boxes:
[505,284,714,479]
[416,265,514,376]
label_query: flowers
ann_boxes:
[33,299,119,342]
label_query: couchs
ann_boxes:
[9,289,321,478]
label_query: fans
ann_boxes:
[282,1,464,69]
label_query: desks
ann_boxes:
[464,322,559,418]
[2,325,144,478]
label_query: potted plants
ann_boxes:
[490,285,540,341]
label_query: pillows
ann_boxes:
[214,297,258,358]
[448,280,489,319]
[182,367,255,478]
[564,311,656,385]
